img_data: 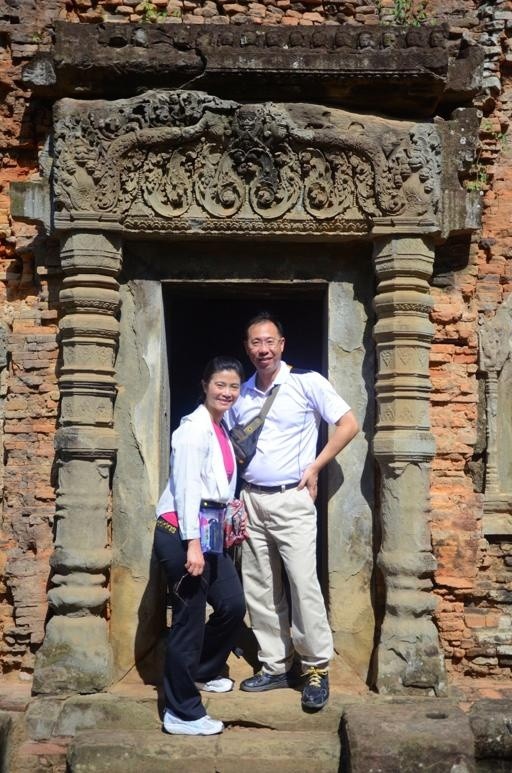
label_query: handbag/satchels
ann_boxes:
[228,416,264,472]
[224,499,251,551]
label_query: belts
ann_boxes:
[243,480,298,493]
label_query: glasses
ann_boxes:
[249,339,281,349]
[173,571,210,610]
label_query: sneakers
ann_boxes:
[195,677,233,693]
[240,665,291,693]
[301,669,330,709]
[163,712,224,737]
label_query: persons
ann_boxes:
[218,307,361,715]
[152,352,250,738]
